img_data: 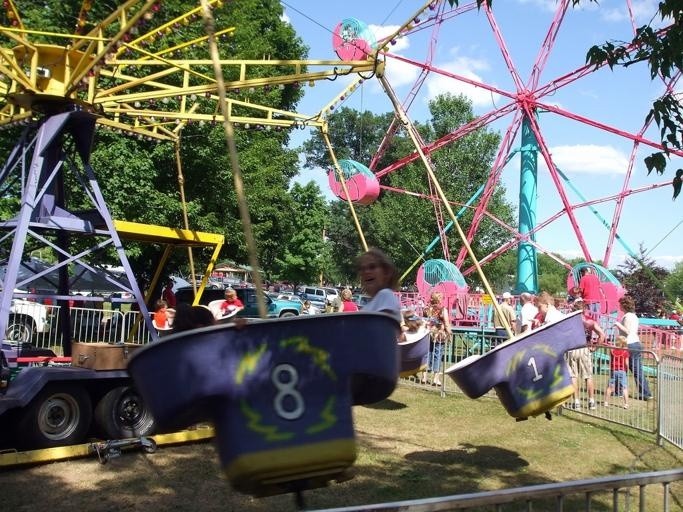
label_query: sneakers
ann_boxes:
[589,400,596,409]
[639,394,651,401]
[572,403,580,409]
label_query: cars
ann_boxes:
[0,297,49,349]
[179,272,373,318]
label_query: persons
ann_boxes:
[161,279,177,309]
[494,287,652,418]
[578,267,605,319]
[218,288,245,318]
[154,299,171,327]
[658,307,682,338]
[298,284,451,390]
[69,294,122,344]
[342,248,400,321]
